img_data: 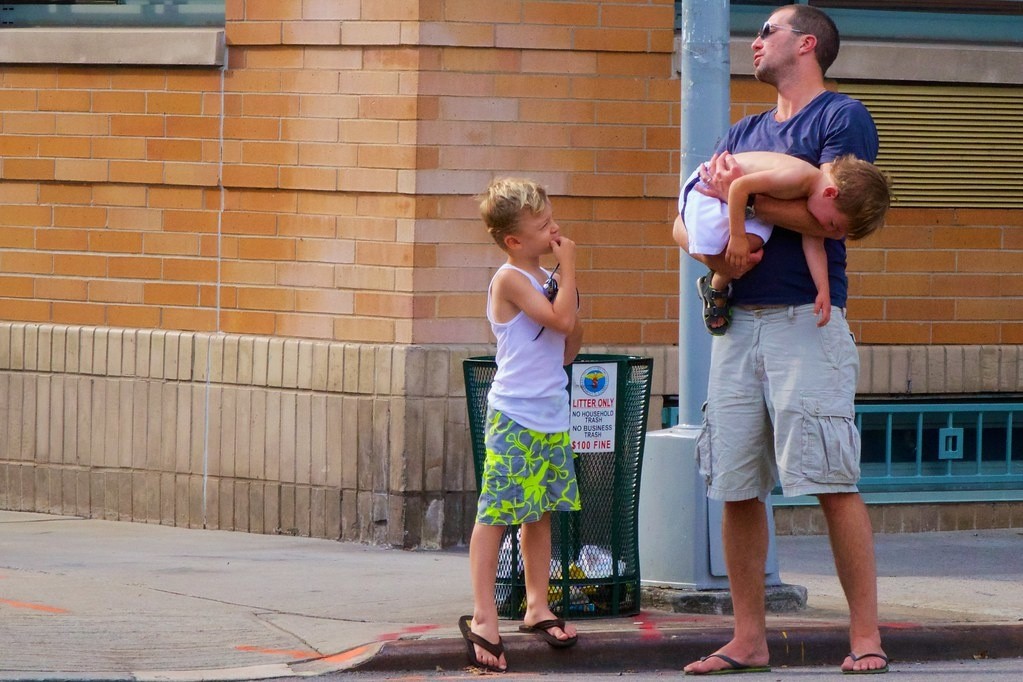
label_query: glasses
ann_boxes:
[755,22,806,39]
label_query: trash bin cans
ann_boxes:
[463,351,656,619]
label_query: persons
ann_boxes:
[677,150,890,337]
[460,180,583,673]
[673,2,890,675]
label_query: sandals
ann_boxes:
[696,270,733,336]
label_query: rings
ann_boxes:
[707,178,712,185]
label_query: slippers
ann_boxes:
[458,614,507,671]
[518,617,578,647]
[685,654,771,675]
[842,652,889,674]
